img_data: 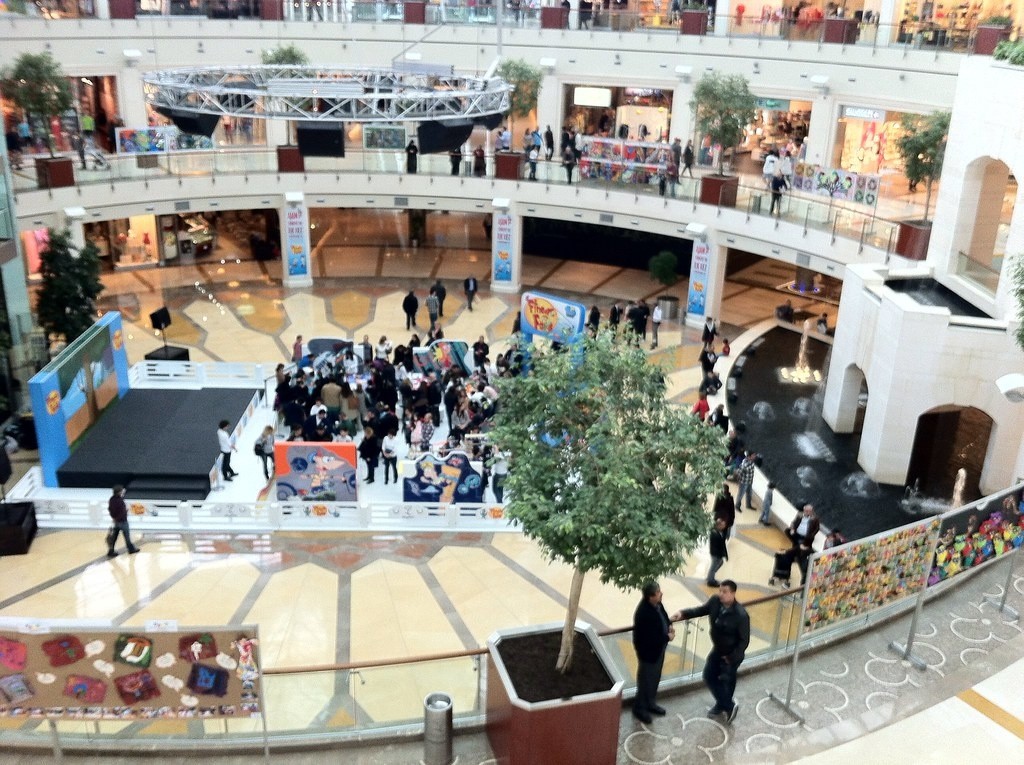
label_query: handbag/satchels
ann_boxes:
[255,444,263,455]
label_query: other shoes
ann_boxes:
[764,524,771,526]
[708,580,720,588]
[225,478,232,481]
[364,477,370,481]
[129,548,140,554]
[395,477,397,482]
[231,473,238,476]
[108,552,118,556]
[632,705,651,724]
[367,480,374,484]
[386,480,388,484]
[708,704,724,714]
[726,700,738,724]
[645,702,666,714]
[747,506,756,510]
[760,519,764,522]
[736,507,742,512]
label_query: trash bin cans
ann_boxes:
[423,693,454,765]
[465,162,471,175]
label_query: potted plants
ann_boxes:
[485,320,733,765]
[975,16,1016,55]
[648,250,680,322]
[494,59,542,179]
[687,71,761,206]
[894,106,953,262]
[0,53,75,188]
[261,43,315,173]
[680,2,709,35]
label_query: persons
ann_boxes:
[735,451,757,513]
[669,580,750,726]
[758,481,776,526]
[589,299,662,350]
[403,273,477,331]
[7,126,19,164]
[406,108,807,215]
[221,115,253,144]
[217,419,238,481]
[707,518,728,586]
[107,486,140,556]
[691,316,744,478]
[81,111,94,138]
[776,299,793,319]
[818,313,832,334]
[483,213,492,239]
[784,504,847,585]
[255,321,523,483]
[561,0,591,31]
[633,580,675,724]
[714,484,735,541]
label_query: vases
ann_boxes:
[259,0,283,20]
[404,2,426,24]
[825,20,858,45]
[540,7,568,30]
[109,0,136,19]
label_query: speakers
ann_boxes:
[150,306,171,330]
[0,447,12,485]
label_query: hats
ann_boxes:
[114,485,123,493]
